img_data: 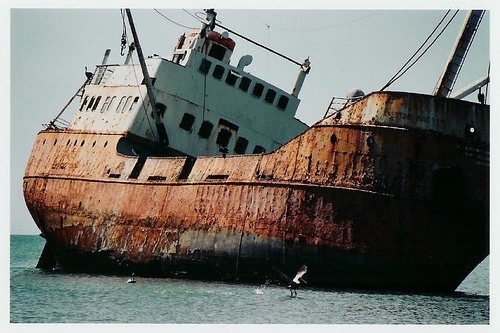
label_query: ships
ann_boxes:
[22,9,490,291]
